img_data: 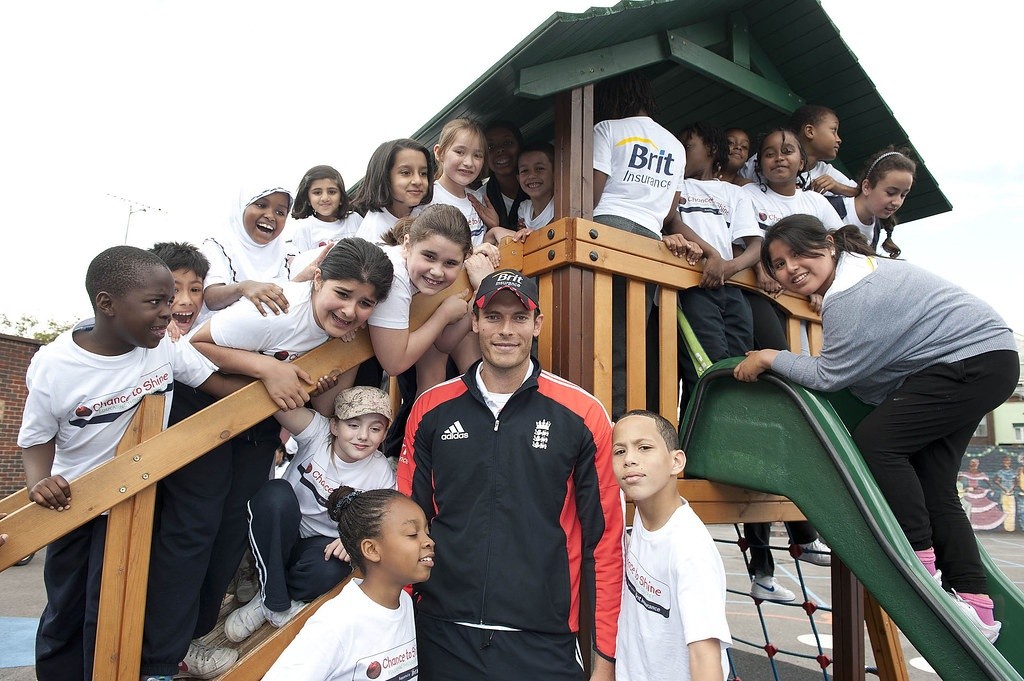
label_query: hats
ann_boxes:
[333,386,394,431]
[474,270,541,317]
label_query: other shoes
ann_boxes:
[942,586,1002,646]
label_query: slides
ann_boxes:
[677,354,1024,680]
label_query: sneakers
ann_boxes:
[267,599,309,629]
[750,576,796,602]
[795,538,831,566]
[237,563,259,603]
[225,591,267,642]
[173,638,238,680]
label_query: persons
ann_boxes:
[398,267,623,681]
[260,487,436,681]
[611,410,733,681]
[17,117,555,681]
[589,89,917,603]
[733,213,1020,647]
